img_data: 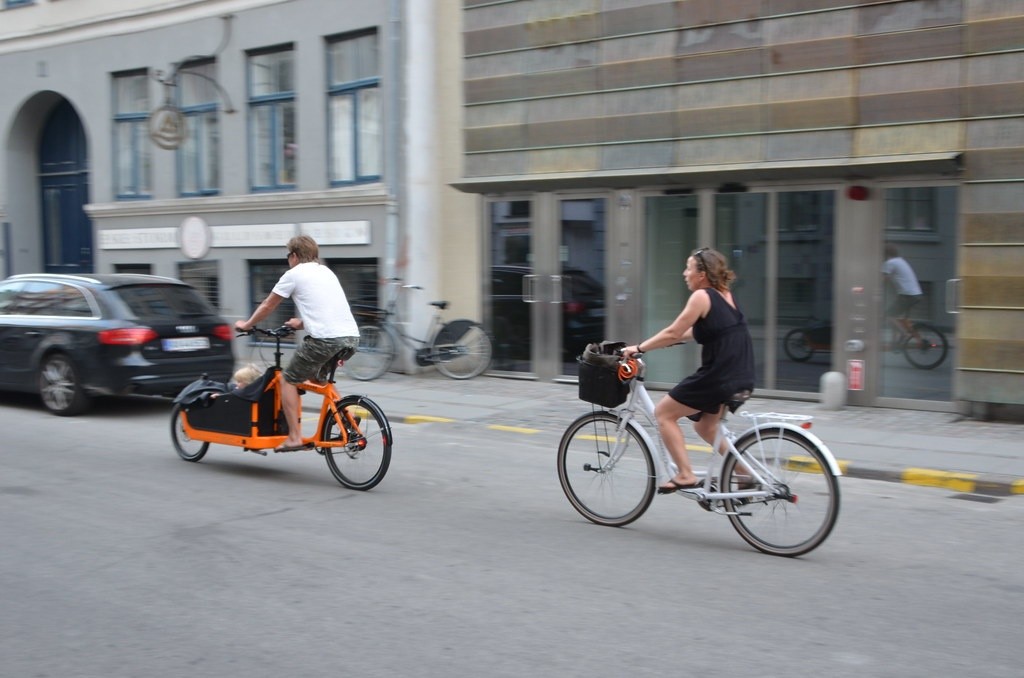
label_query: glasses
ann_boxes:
[694,247,709,271]
[287,250,296,259]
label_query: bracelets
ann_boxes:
[637,343,646,353]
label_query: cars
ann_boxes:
[0,270,235,417]
[490,262,606,358]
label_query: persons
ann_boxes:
[235,236,360,451]
[211,364,262,398]
[621,247,755,496]
[883,244,929,353]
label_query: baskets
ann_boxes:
[576,354,630,408]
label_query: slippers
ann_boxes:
[896,334,912,349]
[341,416,361,435]
[274,442,307,453]
[738,476,765,504]
[658,479,696,494]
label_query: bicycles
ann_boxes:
[557,340,843,557]
[784,300,951,371]
[168,324,395,492]
[344,277,494,382]
